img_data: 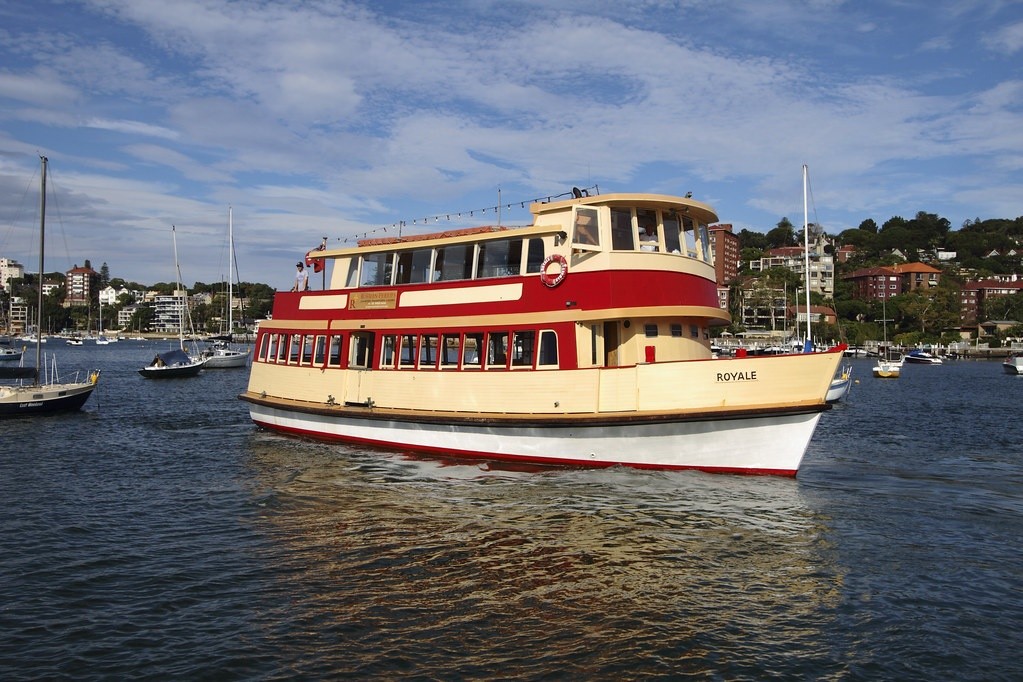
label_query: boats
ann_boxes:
[1003,357,1023,375]
[843,349,867,357]
[0,335,12,343]
[238,183,848,478]
[0,346,23,360]
[96,336,108,345]
[69,333,82,340]
[106,337,118,343]
[730,348,765,355]
[711,345,722,355]
[763,347,789,354]
[66,339,83,346]
[905,353,943,366]
[877,352,905,368]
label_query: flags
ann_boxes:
[305,246,325,273]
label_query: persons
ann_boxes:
[292,262,309,292]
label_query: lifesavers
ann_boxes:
[539,254,569,287]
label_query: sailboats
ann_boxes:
[873,274,900,378]
[0,156,101,415]
[137,317,148,341]
[188,207,251,368]
[129,323,137,339]
[803,164,852,403]
[137,226,211,378]
[14,306,47,343]
